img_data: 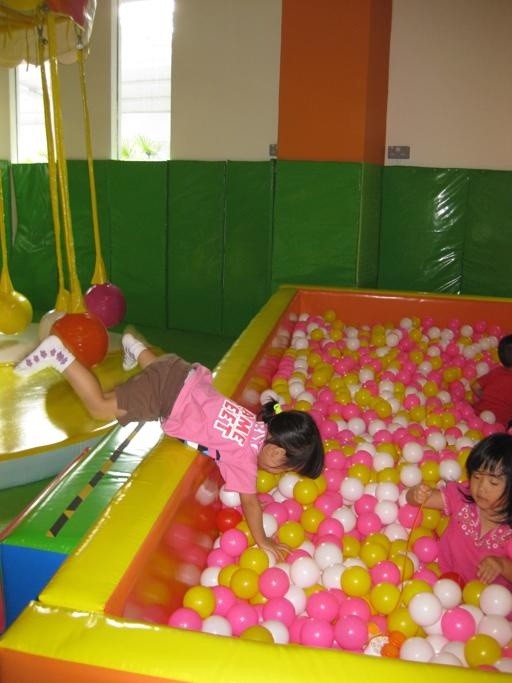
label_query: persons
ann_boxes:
[471,335,511,430]
[13,333,324,562]
[405,433,511,591]
